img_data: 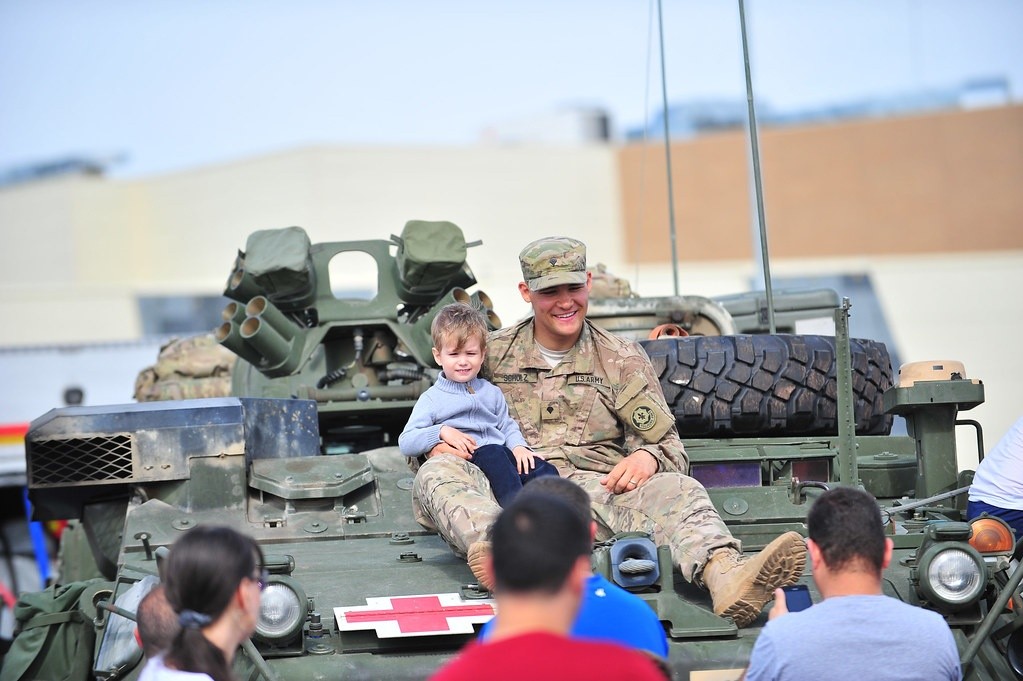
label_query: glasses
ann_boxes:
[253,573,268,592]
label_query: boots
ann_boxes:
[466,540,498,600]
[700,531,807,629]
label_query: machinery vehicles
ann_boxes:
[20,1,1023,681]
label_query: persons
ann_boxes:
[966,417,1023,563]
[427,474,672,681]
[736,487,963,681]
[134,524,267,681]
[399,301,559,511]
[403,235,807,630]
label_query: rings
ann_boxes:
[629,480,637,485]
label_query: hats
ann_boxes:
[519,237,587,292]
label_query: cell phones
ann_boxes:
[780,584,812,612]
